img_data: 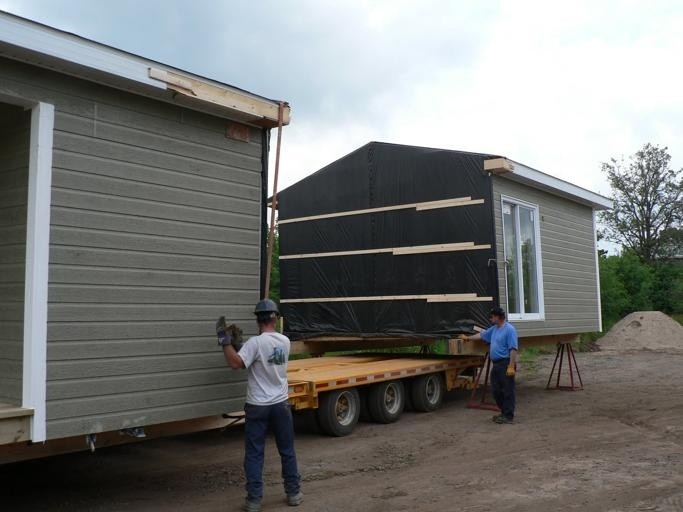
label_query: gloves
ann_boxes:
[231,323,243,345]
[505,364,516,377]
[215,315,233,346]
[458,334,470,341]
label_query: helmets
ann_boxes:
[253,299,280,316]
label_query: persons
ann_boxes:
[456,307,519,424]
[214,297,305,512]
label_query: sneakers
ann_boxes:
[286,490,306,507]
[239,498,263,512]
[492,414,516,425]
